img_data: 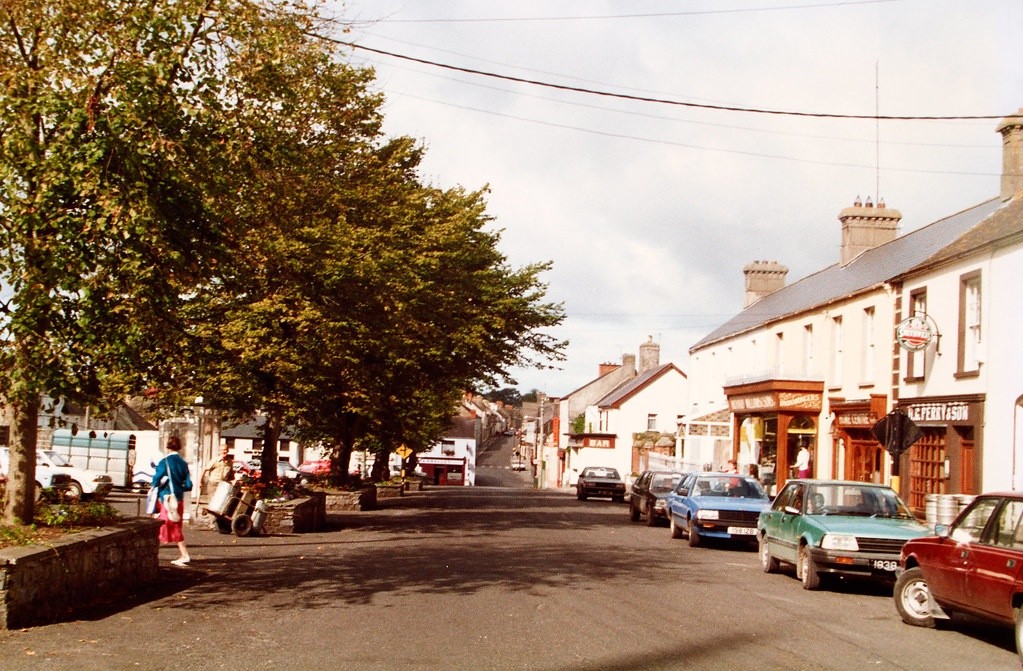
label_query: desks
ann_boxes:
[112,484,150,493]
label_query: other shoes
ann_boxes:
[171,555,190,564]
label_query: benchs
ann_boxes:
[93,496,142,517]
[103,491,148,498]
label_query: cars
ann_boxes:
[895,491,1023,665]
[628,469,690,525]
[663,471,774,547]
[576,467,626,501]
[758,478,942,596]
[0,444,114,503]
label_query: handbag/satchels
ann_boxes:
[145,487,158,514]
[164,493,180,522]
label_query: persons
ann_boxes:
[800,493,830,517]
[198,443,235,530]
[149,436,192,566]
[789,442,812,480]
[744,462,761,488]
[720,459,740,496]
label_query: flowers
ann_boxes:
[240,468,298,500]
[312,464,363,490]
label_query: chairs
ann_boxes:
[811,492,829,515]
[730,486,749,497]
[697,481,715,496]
